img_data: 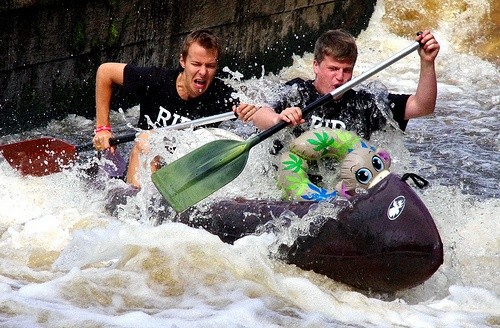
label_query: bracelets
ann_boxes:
[95,125,111,133]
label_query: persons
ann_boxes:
[92,30,259,189]
[253,29,440,185]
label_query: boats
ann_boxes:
[65,119,444,294]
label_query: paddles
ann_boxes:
[0,97,242,177]
[151,31,429,212]
